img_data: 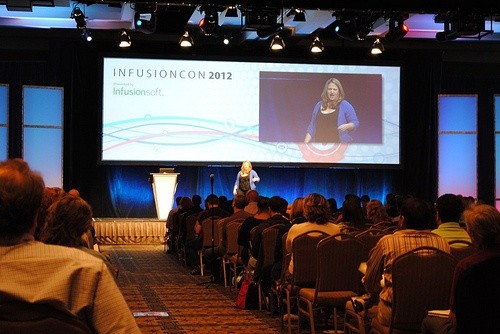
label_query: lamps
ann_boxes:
[70,2,499,55]
[0,0,56,11]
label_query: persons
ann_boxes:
[304,78,360,143]
[233,162,260,195]
[0,159,141,334]
[421,204,500,334]
[34,187,476,310]
[352,198,450,327]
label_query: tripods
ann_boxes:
[195,174,218,289]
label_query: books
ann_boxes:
[428,310,450,319]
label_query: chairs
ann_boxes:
[164,221,500,334]
[0,299,98,334]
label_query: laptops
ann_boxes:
[160,168,174,173]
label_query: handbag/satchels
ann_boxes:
[237,274,258,310]
